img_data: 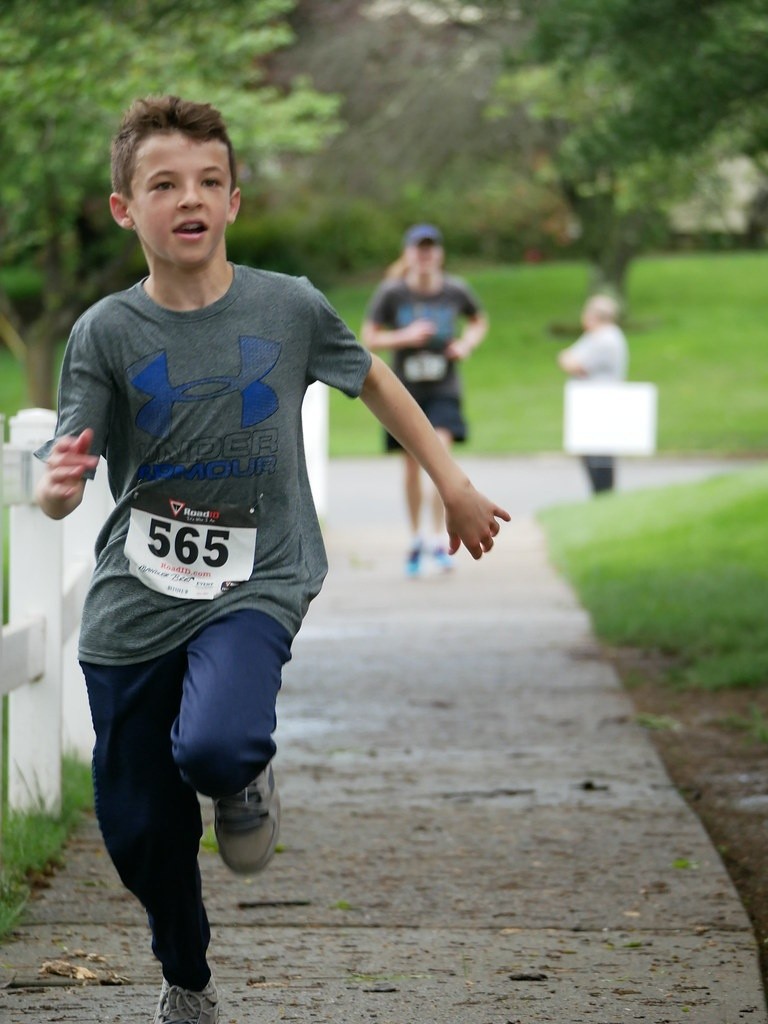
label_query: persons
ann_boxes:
[35,95,512,1024]
[559,294,629,492]
[360,222,488,577]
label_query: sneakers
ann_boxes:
[212,762,283,874]
[404,541,423,576]
[154,973,219,1024]
[431,546,454,569]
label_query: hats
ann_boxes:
[405,223,442,246]
